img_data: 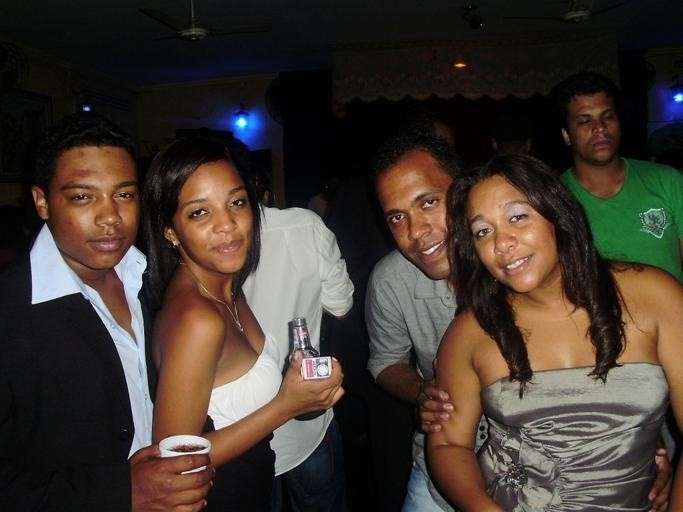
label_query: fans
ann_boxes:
[141,0,231,47]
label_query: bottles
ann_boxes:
[285,317,326,420]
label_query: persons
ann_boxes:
[425,155,682,512]
[363,142,471,512]
[555,74,682,273]
[151,134,345,512]
[239,142,352,512]
[0,116,213,512]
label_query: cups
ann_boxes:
[158,433,213,475]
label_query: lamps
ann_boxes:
[233,102,249,128]
